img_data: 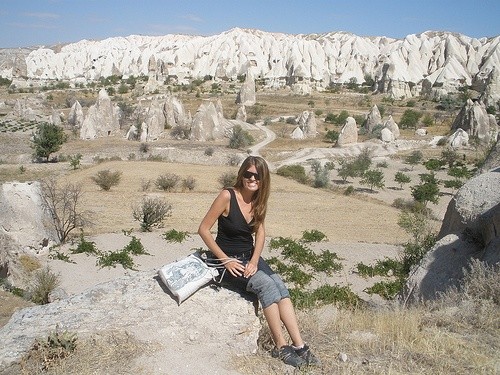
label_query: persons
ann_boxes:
[197,155,321,369]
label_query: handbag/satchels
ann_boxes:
[158,254,237,304]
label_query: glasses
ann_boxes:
[243,171,260,180]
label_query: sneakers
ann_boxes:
[272,344,320,368]
